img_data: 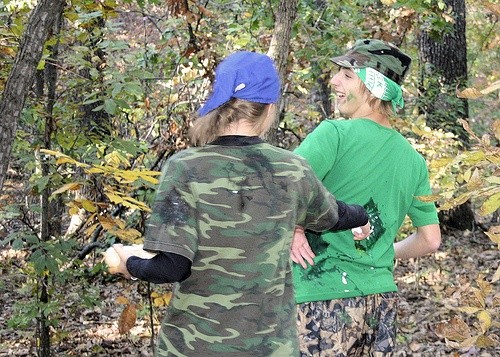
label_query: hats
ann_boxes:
[330,39,412,84]
